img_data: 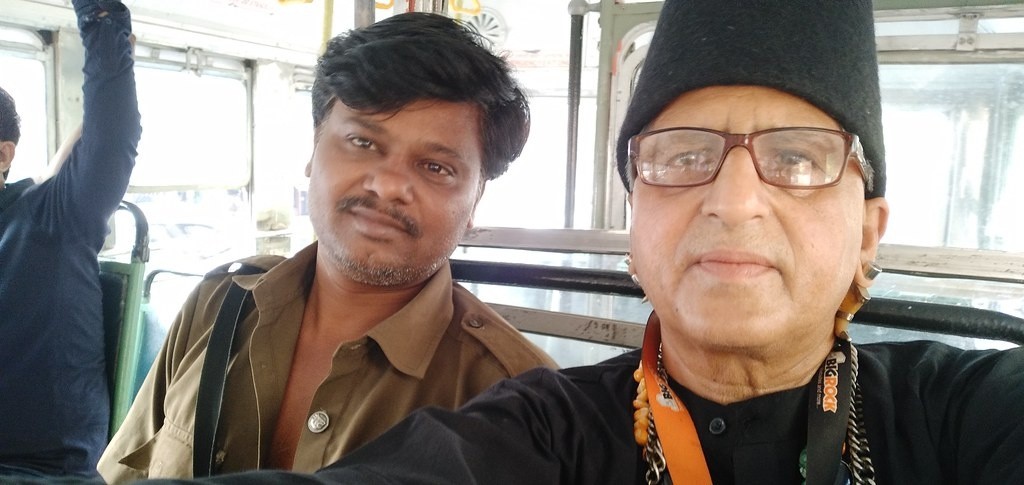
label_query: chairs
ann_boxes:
[96,201,150,458]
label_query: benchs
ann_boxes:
[447,229,1024,352]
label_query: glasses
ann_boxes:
[628,127,874,190]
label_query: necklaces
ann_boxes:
[632,338,876,485]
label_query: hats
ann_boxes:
[616,0,887,202]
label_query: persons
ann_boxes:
[95,12,561,485]
[0,0,144,485]
[133,0,1024,485]
